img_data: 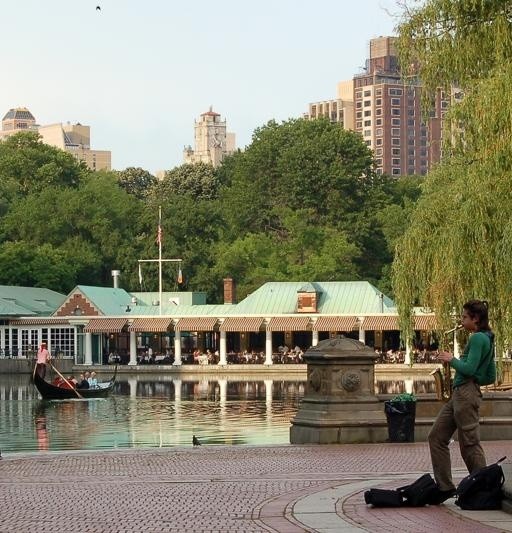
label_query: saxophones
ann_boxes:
[429,325,464,402]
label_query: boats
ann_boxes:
[33,364,122,399]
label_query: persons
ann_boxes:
[36,342,53,380]
[376,347,424,364]
[193,349,220,364]
[51,371,98,390]
[109,350,121,362]
[138,345,173,364]
[428,300,496,492]
[277,345,304,363]
[228,348,265,363]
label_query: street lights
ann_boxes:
[111,268,121,287]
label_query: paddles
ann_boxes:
[48,362,83,398]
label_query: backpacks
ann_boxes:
[455,456,507,509]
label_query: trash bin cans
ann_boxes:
[384,400,416,443]
